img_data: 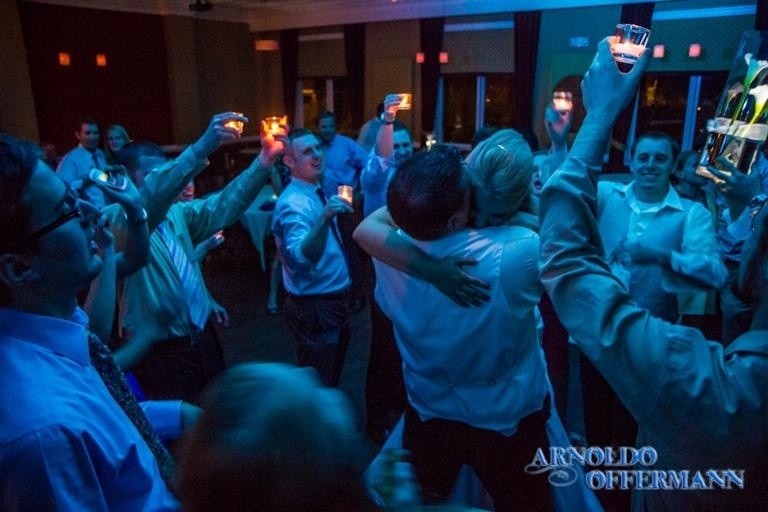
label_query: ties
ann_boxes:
[87,332,182,501]
[157,223,210,332]
[316,186,350,267]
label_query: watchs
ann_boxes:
[380,111,396,124]
[750,192,767,210]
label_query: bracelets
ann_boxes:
[122,208,148,224]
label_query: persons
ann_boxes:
[351,129,603,511]
[102,123,133,167]
[179,178,225,268]
[544,102,728,512]
[360,93,413,453]
[115,112,291,407]
[371,145,558,512]
[55,113,129,346]
[266,158,291,315]
[0,132,184,512]
[536,38,768,512]
[270,129,356,388]
[529,148,571,415]
[671,151,708,208]
[705,157,768,215]
[316,111,370,315]
[77,164,152,345]
[355,103,385,151]
[174,362,390,512]
[708,151,768,347]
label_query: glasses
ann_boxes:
[28,178,83,240]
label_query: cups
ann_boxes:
[396,93,412,110]
[552,92,573,112]
[337,186,352,203]
[226,112,245,134]
[266,115,289,138]
[613,23,651,62]
